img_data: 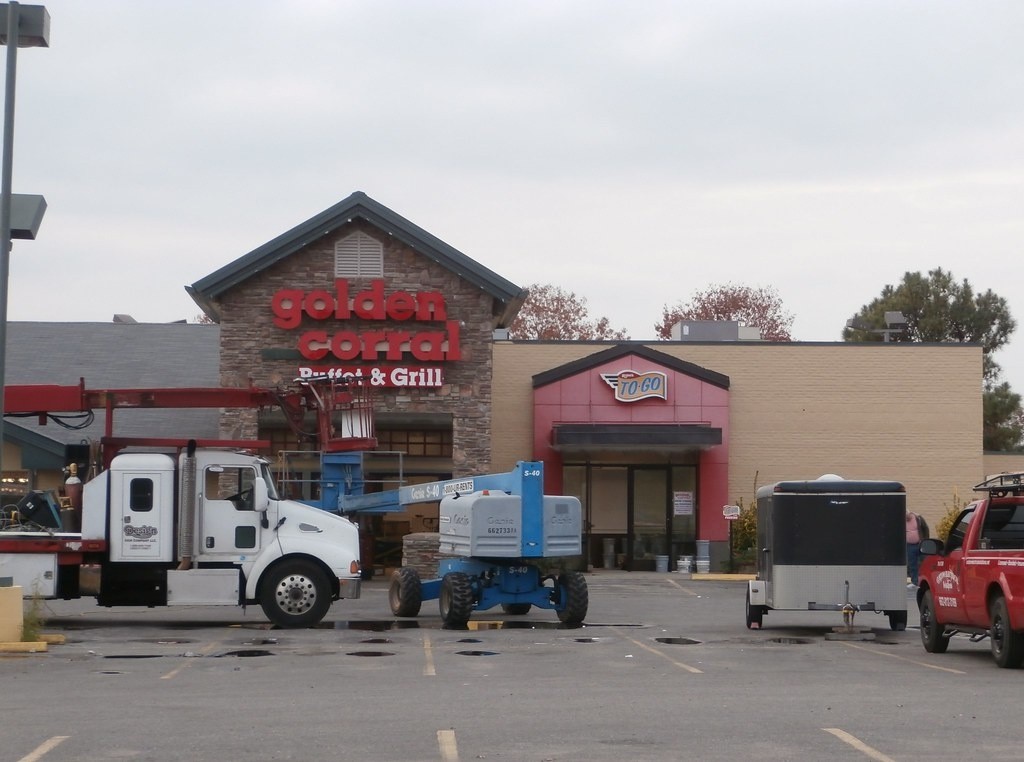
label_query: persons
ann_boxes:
[906,510,930,588]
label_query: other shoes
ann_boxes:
[907,583,918,589]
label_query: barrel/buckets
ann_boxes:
[676,540,711,573]
[654,555,669,572]
[601,537,627,570]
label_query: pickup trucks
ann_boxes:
[916,471,1024,669]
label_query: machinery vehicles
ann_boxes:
[0,376,379,632]
[297,449,591,629]
